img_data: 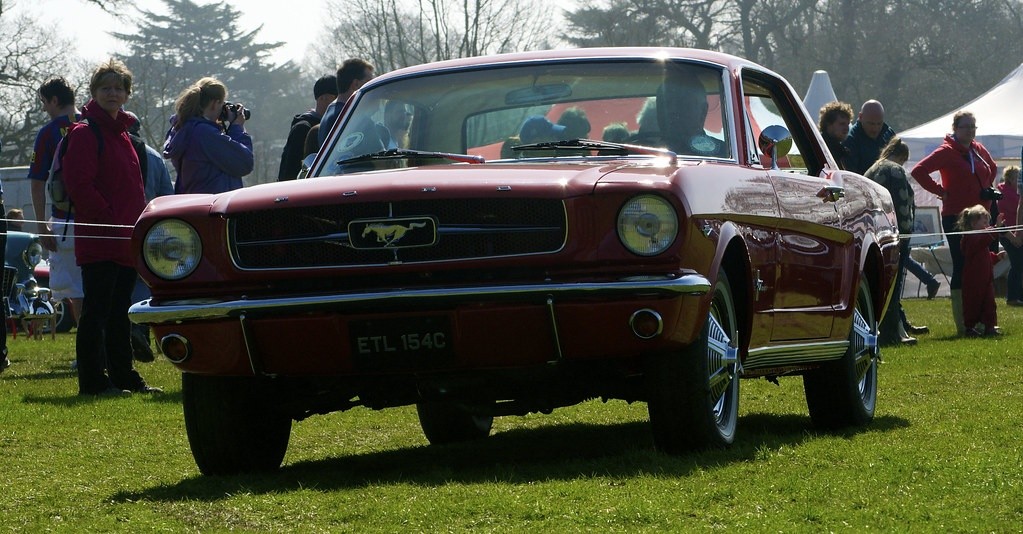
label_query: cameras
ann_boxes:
[219,101,250,120]
[981,187,1003,200]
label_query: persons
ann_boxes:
[6,209,24,232]
[954,204,1006,338]
[997,146,1023,306]
[26,57,175,397]
[911,111,997,334]
[276,58,413,182]
[817,99,941,347]
[165,77,254,194]
[499,97,658,161]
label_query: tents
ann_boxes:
[894,63,1023,299]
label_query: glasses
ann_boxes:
[957,125,978,131]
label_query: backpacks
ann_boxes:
[47,116,104,214]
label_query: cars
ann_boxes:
[125,47,904,483]
[26,265,86,334]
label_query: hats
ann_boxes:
[519,113,565,144]
[313,73,340,97]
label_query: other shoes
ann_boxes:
[927,280,941,300]
[899,335,917,345]
[983,329,999,337]
[965,328,981,336]
[0,355,11,373]
[132,329,154,363]
[909,325,930,334]
[104,386,130,395]
[1007,298,1023,307]
[134,382,165,393]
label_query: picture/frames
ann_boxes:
[909,206,944,246]
